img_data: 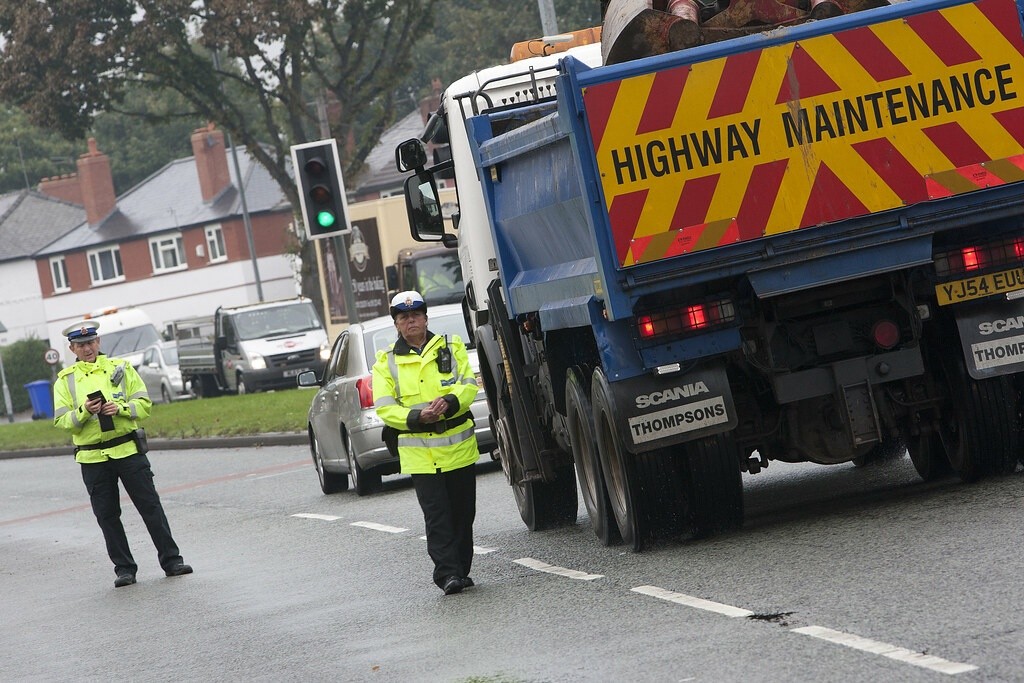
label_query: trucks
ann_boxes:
[386,246,465,304]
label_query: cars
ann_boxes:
[136,341,195,403]
[308,302,497,498]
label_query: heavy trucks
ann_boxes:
[392,0,1024,556]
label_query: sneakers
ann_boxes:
[461,576,473,586]
[114,573,136,587]
[444,575,463,595]
[166,565,193,576]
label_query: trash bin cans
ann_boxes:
[24,381,54,420]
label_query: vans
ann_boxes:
[177,296,333,399]
[81,305,165,371]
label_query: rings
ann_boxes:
[441,405,444,407]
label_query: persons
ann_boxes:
[372,291,480,595]
[53,320,194,587]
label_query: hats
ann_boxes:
[61,320,100,342]
[389,291,428,316]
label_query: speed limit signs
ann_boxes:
[43,350,60,364]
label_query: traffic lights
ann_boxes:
[289,139,351,240]
